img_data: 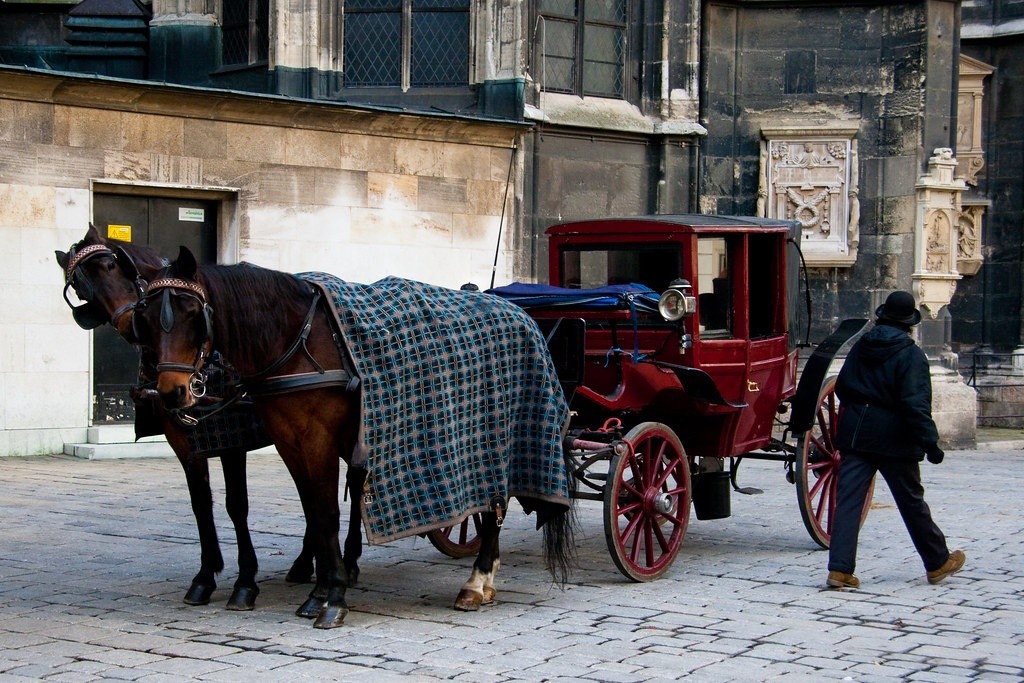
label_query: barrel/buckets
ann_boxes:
[691,456,731,521]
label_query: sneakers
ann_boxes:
[926,550,966,584]
[826,570,861,588]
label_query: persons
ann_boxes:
[827,290,966,585]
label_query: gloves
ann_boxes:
[928,444,944,464]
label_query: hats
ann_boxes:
[875,292,921,326]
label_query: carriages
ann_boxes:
[53,212,872,632]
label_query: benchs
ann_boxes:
[528,309,678,391]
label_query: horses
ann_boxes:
[54,220,370,612]
[131,244,584,629]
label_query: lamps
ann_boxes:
[668,277,693,354]
